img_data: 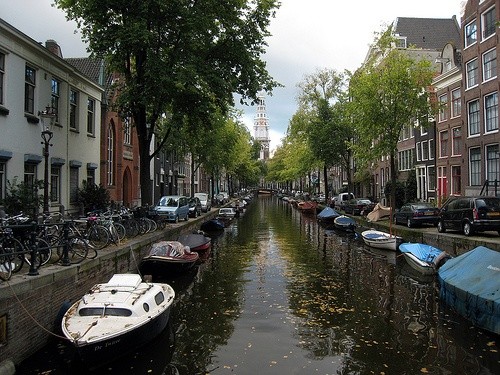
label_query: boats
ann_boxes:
[137,240,199,278]
[437,245,500,335]
[201,219,224,235]
[219,207,237,218]
[318,207,341,223]
[333,214,357,231]
[276,193,316,212]
[367,201,392,221]
[176,234,213,253]
[229,193,254,212]
[61,274,176,357]
[399,242,455,277]
[361,230,403,250]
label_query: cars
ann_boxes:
[186,197,203,218]
[432,197,500,236]
[154,195,189,223]
[345,198,375,216]
[219,192,231,203]
[194,192,212,213]
[317,194,326,203]
[214,194,226,206]
[330,196,336,206]
[392,202,441,228]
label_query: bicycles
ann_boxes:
[0,201,166,282]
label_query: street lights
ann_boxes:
[34,104,60,252]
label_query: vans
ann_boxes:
[335,193,356,209]
